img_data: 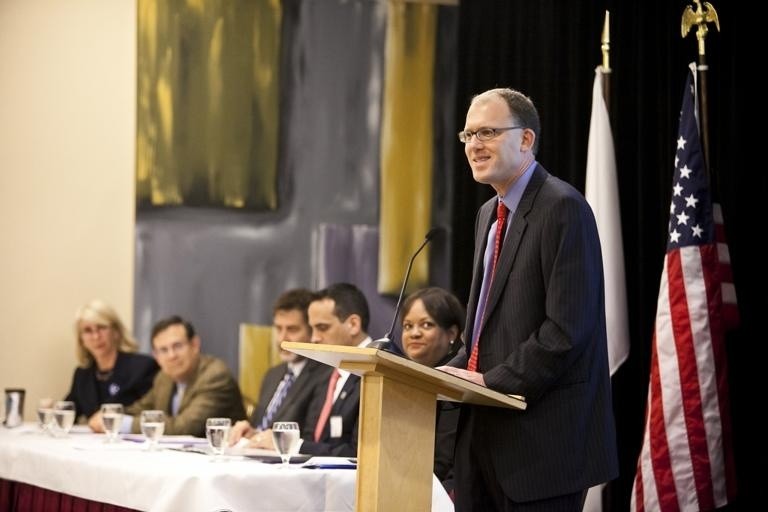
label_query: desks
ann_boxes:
[1,423,457,511]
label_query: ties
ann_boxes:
[314,368,341,443]
[467,202,509,371]
[257,368,293,433]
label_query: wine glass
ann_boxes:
[140,409,165,451]
[99,403,124,445]
[35,399,77,442]
[272,421,300,472]
[205,417,232,463]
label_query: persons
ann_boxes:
[250,287,316,429]
[452,87,619,511]
[86,315,247,441]
[227,282,373,457]
[401,287,468,479]
[62,298,160,426]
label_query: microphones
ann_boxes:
[366,227,439,361]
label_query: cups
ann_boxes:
[3,388,26,428]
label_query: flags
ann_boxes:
[580,64,633,378]
[628,58,739,511]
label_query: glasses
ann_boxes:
[458,125,520,143]
